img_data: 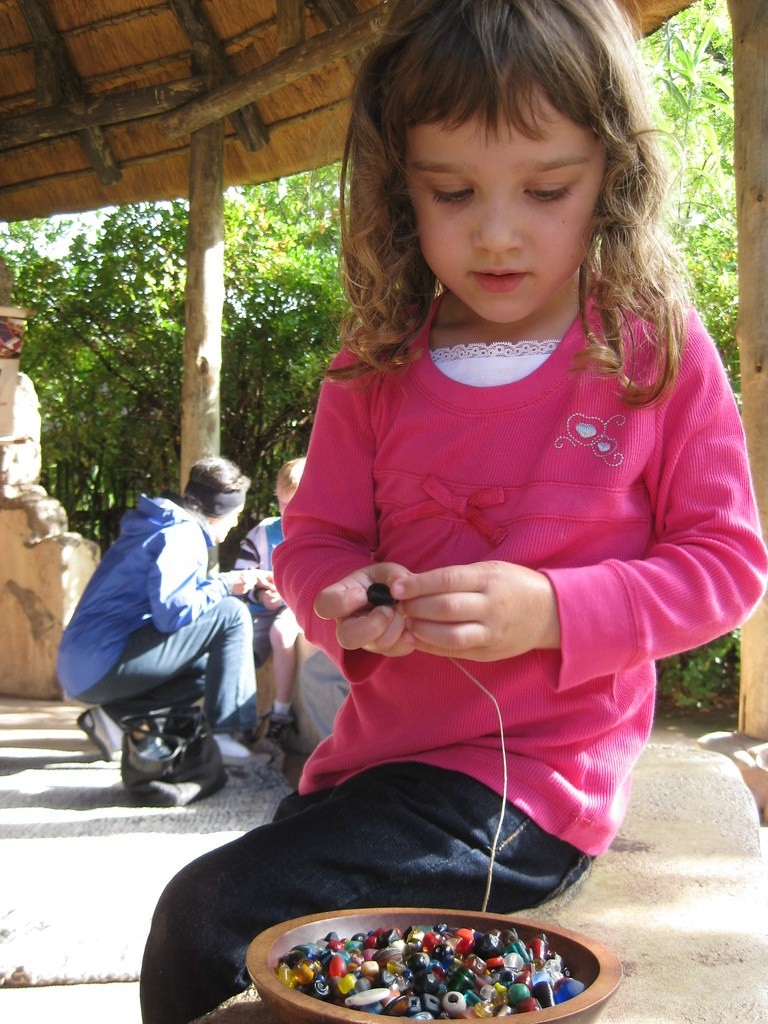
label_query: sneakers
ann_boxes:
[77,706,124,761]
[257,709,300,751]
[233,728,258,745]
[208,731,272,777]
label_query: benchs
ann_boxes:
[199,624,768,1023]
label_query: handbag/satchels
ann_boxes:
[120,704,229,807]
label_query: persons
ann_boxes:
[198,457,313,744]
[140,1,766,1023]
[54,453,278,768]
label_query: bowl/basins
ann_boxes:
[242,908,624,1023]
